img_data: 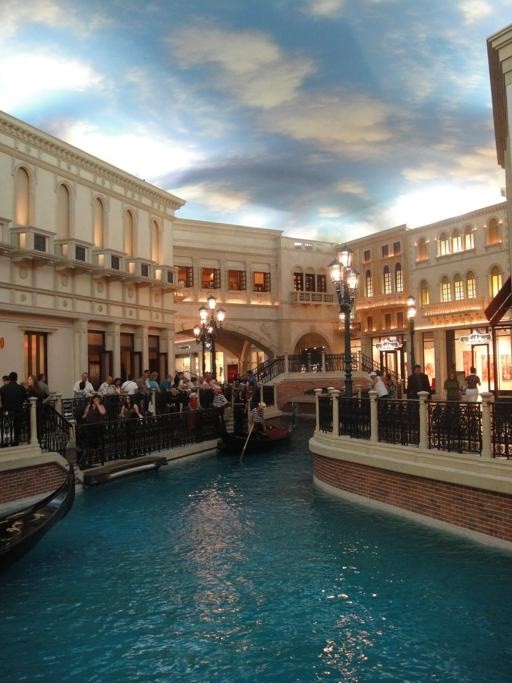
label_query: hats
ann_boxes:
[258,401,266,409]
[368,371,378,377]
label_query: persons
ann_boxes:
[406,364,433,430]
[366,366,397,412]
[0,365,268,467]
[443,370,461,413]
[464,366,483,412]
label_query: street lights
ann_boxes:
[328,243,360,395]
[403,293,416,373]
[190,324,212,375]
[200,294,226,380]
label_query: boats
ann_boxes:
[0,462,76,568]
[215,403,298,451]
